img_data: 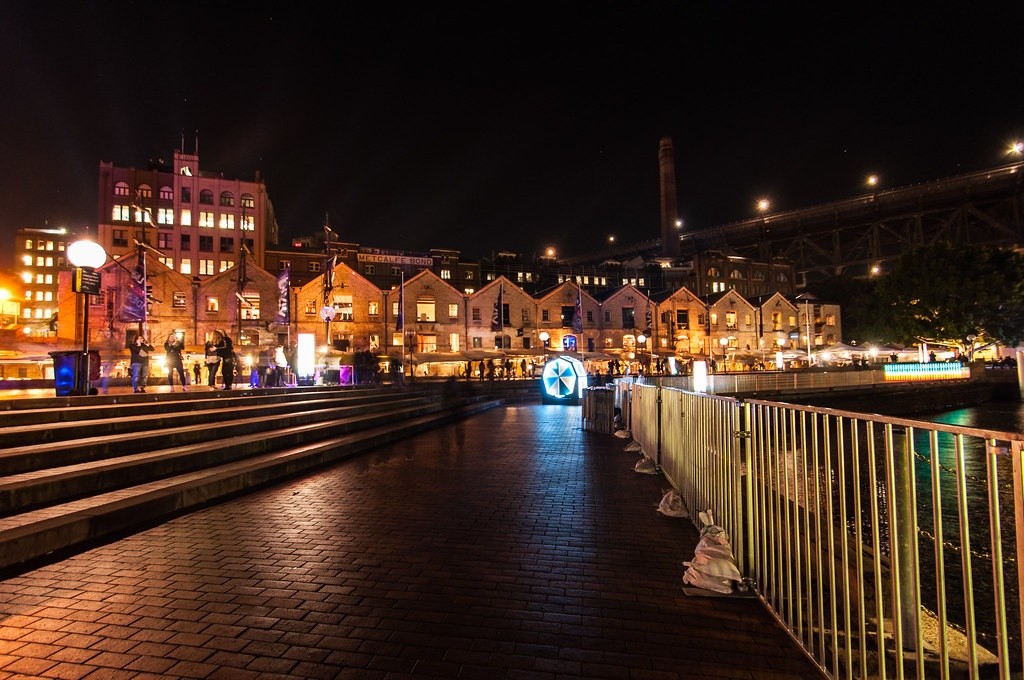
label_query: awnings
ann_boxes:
[415,348,611,362]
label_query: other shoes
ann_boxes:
[171,386,174,392]
[182,386,190,391]
[134,387,146,393]
[209,382,232,391]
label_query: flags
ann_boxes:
[396,280,403,330]
[646,298,651,329]
[573,291,583,333]
[490,286,503,332]
[119,254,146,323]
[275,268,290,325]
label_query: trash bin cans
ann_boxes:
[258,366,270,387]
[50,348,100,395]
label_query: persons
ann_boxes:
[205,330,234,390]
[889,352,898,362]
[466,358,535,380]
[193,362,201,385]
[130,335,154,393]
[164,334,188,392]
[251,344,289,388]
[929,351,936,362]
[608,359,620,374]
